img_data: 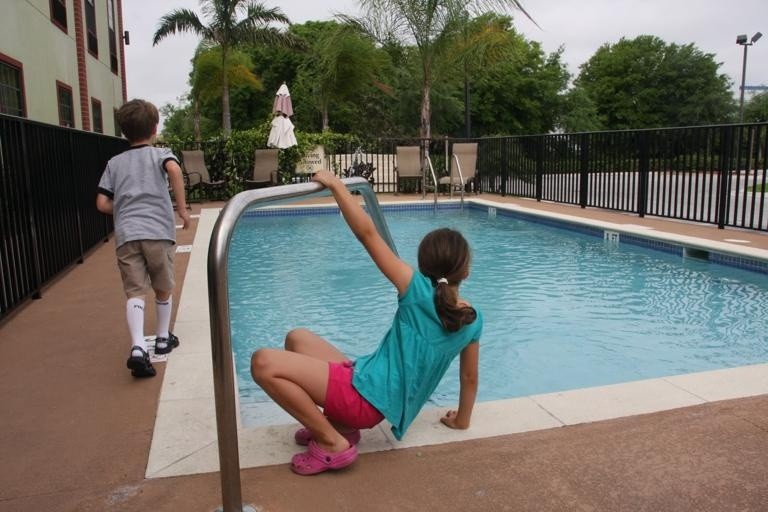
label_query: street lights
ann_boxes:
[735,31,763,113]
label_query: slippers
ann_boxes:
[291,439,359,477]
[295,427,361,446]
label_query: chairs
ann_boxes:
[393,142,479,197]
[168,148,281,210]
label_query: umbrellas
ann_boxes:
[267,82,299,149]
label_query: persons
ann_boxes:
[250,168,483,474]
[96,99,190,379]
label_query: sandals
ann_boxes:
[154,331,178,355]
[126,345,157,377]
